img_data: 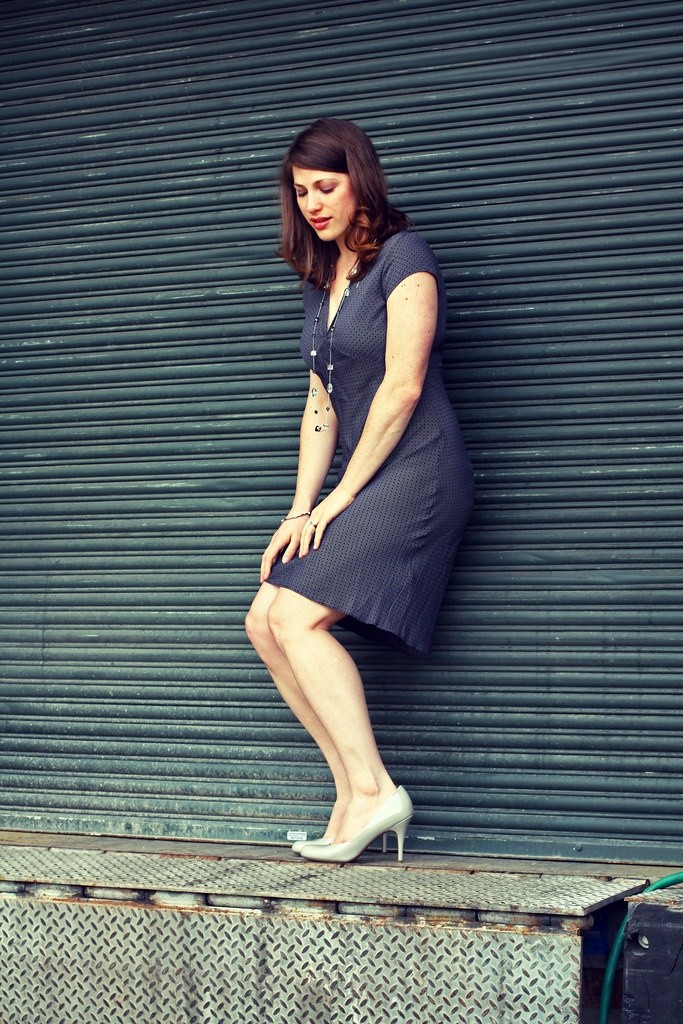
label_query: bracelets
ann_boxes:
[280,511,311,523]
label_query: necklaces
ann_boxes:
[310,234,378,433]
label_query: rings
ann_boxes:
[308,521,314,525]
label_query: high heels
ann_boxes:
[292,831,391,854]
[300,784,413,863]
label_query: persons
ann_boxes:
[244,118,477,863]
